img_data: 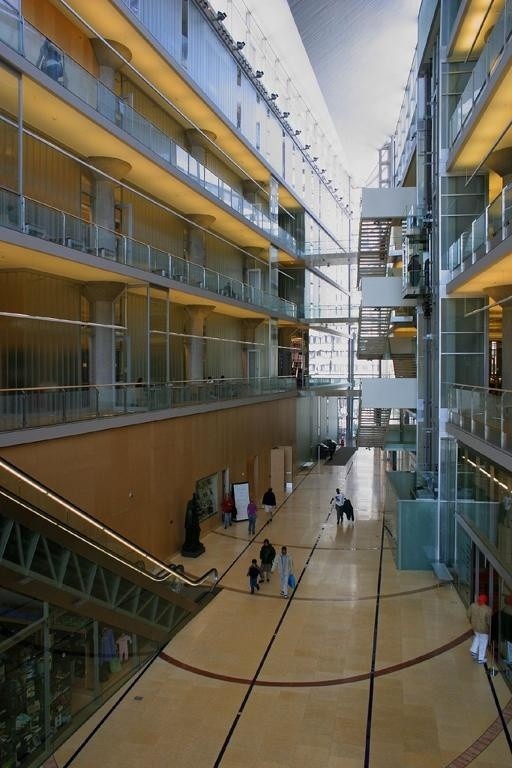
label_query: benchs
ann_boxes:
[430,563,454,592]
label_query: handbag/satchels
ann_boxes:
[288,574,297,589]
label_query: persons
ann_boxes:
[261,487,277,524]
[221,492,235,530]
[224,282,235,299]
[246,497,258,535]
[247,558,264,594]
[326,441,333,461]
[271,545,295,599]
[330,487,348,525]
[499,491,512,528]
[220,376,225,383]
[205,376,214,384]
[185,492,205,551]
[409,254,422,287]
[134,377,144,388]
[465,594,493,665]
[35,37,62,83]
[258,538,275,584]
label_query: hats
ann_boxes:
[478,594,487,604]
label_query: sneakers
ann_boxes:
[248,531,255,534]
[267,519,273,524]
[472,653,488,665]
[250,577,288,599]
[224,523,233,529]
